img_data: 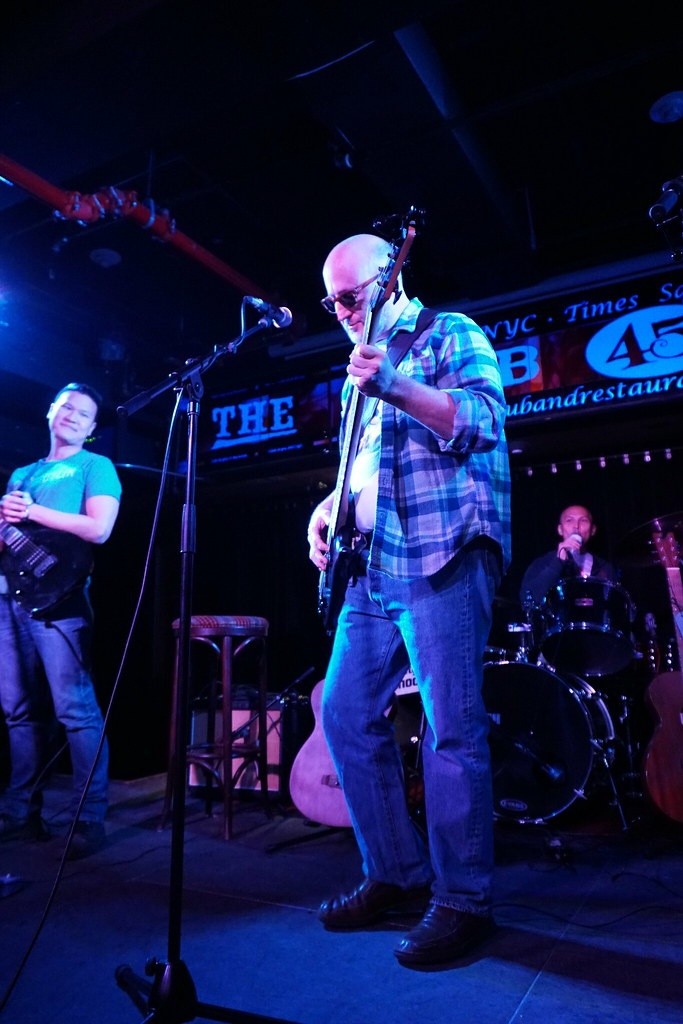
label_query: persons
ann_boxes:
[520,505,625,610]
[0,382,125,860]
[306,233,513,963]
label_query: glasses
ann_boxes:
[321,274,382,313]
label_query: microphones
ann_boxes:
[244,296,293,328]
[231,726,248,738]
[567,534,582,559]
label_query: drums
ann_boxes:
[423,659,615,822]
[532,577,638,676]
[484,647,507,659]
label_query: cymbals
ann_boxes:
[629,511,674,536]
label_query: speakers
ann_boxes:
[184,696,315,800]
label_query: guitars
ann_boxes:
[642,531,683,821]
[0,518,91,621]
[316,223,417,626]
[288,680,355,828]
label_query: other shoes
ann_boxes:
[0,812,42,840]
[62,820,106,846]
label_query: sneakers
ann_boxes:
[392,904,498,964]
[318,878,436,926]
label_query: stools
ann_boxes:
[158,615,271,841]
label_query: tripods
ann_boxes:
[112,307,296,1024]
